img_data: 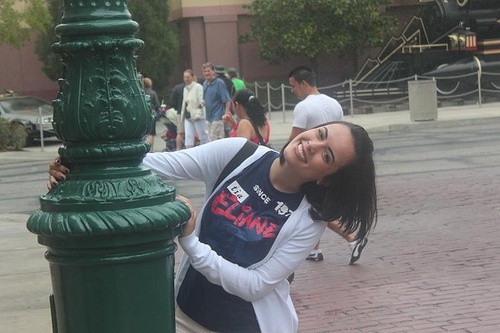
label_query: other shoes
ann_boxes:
[348,236,368,265]
[305,251,323,260]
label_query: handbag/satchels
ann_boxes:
[190,104,207,119]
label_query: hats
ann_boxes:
[215,66,224,73]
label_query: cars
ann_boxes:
[0,96,57,149]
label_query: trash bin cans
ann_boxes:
[408,80,438,122]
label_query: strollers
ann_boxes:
[158,108,201,151]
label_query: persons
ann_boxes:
[47,121,379,333]
[289,66,370,265]
[222,88,270,145]
[142,62,246,151]
